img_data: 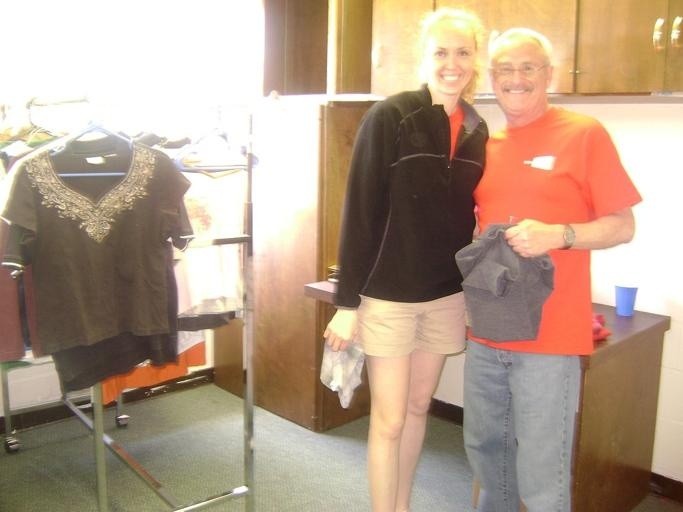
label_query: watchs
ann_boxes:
[559,222,577,249]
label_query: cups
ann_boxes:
[615,285,638,316]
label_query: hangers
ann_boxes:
[0,97,259,181]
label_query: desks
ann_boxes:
[304,279,671,512]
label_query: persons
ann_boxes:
[319,8,490,512]
[460,26,644,511]
